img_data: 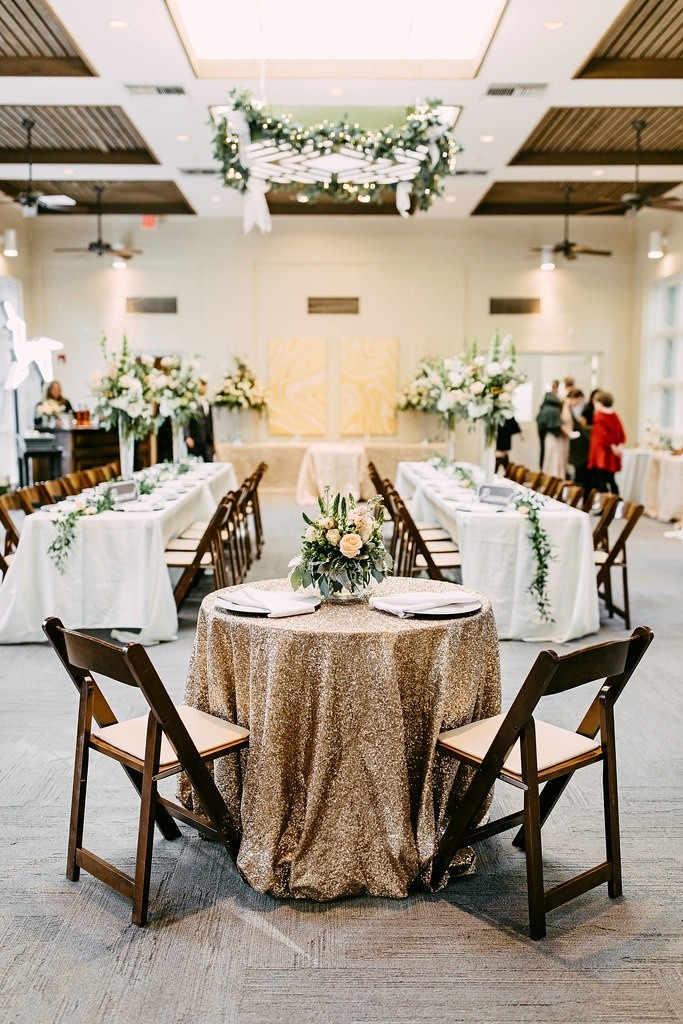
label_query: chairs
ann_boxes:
[164,462,270,612]
[41,617,250,928]
[430,625,655,940]
[504,461,643,629]
[0,461,121,578]
[367,461,461,582]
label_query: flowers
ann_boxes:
[87,330,270,439]
[396,329,528,446]
[290,485,394,597]
[47,456,205,570]
[433,453,556,623]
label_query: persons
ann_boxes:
[495,416,525,474]
[184,379,218,463]
[34,381,72,425]
[537,377,626,517]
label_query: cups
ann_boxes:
[61,416,74,431]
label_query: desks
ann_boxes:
[614,446,683,522]
[297,444,370,504]
[0,461,241,647]
[397,461,600,643]
[214,408,269,443]
[174,577,503,901]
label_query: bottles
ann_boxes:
[76,402,90,426]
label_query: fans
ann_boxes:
[0,119,76,219]
[575,121,683,213]
[525,186,614,258]
[55,186,144,262]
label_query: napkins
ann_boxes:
[420,465,564,514]
[369,589,478,619]
[42,463,221,515]
[215,586,315,618]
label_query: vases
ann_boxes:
[443,415,497,473]
[318,570,373,604]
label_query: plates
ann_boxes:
[39,461,227,511]
[403,601,482,614]
[214,593,322,613]
[410,459,505,512]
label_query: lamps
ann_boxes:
[647,230,667,258]
[541,245,556,270]
[0,228,19,257]
[209,44,455,216]
[109,243,129,268]
